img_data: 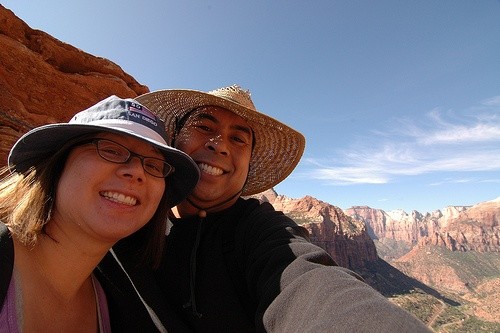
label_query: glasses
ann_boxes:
[67,138,175,178]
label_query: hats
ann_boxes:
[8,94,201,213]
[135,84,306,197]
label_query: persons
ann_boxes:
[132,86,435,333]
[0,94,202,333]
